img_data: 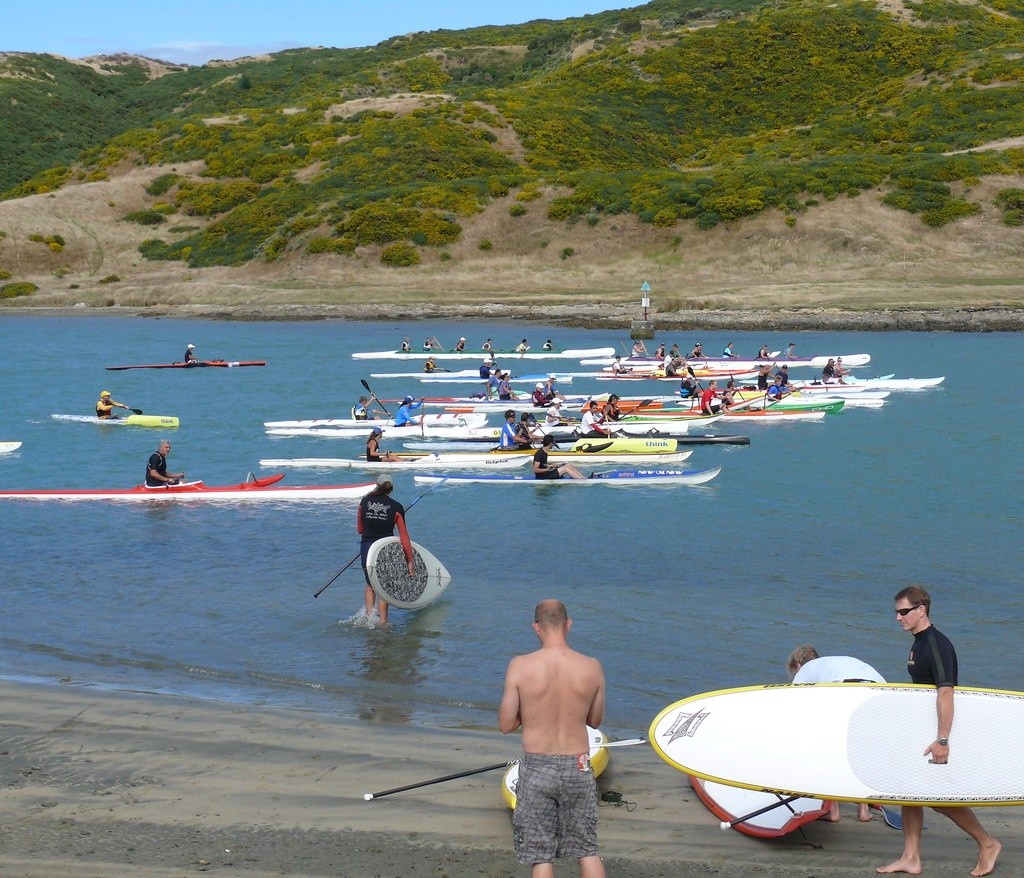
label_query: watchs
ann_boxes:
[935,738,949,746]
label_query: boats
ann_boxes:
[413,466,723,487]
[263,343,944,476]
[0,474,377,502]
[1,441,22,453]
[51,412,179,428]
[105,360,266,370]
[365,537,450,611]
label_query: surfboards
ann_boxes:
[687,774,832,838]
[647,686,1024,806]
[500,723,607,812]
[365,537,451,610]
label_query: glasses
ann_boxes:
[636,343,638,344]
[895,603,927,615]
[831,361,834,363]
[765,346,767,347]
[106,395,109,396]
[506,376,509,378]
[511,416,515,418]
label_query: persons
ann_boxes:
[145,440,186,486]
[394,395,424,427]
[184,344,200,363]
[455,337,466,352]
[423,336,441,352]
[533,435,598,480]
[424,356,444,373]
[366,427,404,462]
[357,474,414,623]
[96,390,129,420]
[875,586,1001,878]
[786,644,888,822]
[352,394,390,420]
[499,599,606,878]
[401,337,412,353]
[479,337,851,451]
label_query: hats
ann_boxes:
[377,474,393,487]
[674,344,678,348]
[547,340,551,343]
[536,383,545,388]
[635,341,639,344]
[459,337,466,340]
[550,373,556,379]
[373,427,385,435]
[405,395,415,403]
[188,344,195,348]
[782,365,787,368]
[615,355,621,358]
[552,397,563,404]
[695,342,702,347]
[484,358,493,363]
[829,360,836,363]
[687,374,692,379]
[101,391,111,398]
[661,343,665,346]
[789,342,794,346]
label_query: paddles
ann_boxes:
[421,398,425,440]
[687,366,705,393]
[718,794,800,832]
[115,404,142,414]
[538,426,560,450]
[720,388,738,410]
[606,415,611,440]
[313,474,450,597]
[433,336,445,353]
[360,377,392,419]
[619,399,652,420]
[363,737,647,802]
[641,341,649,357]
